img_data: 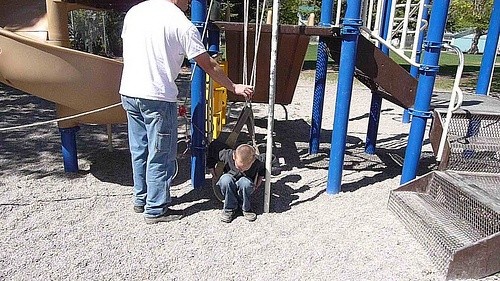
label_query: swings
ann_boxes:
[211,0,268,203]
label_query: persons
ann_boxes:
[119,0,257,226]
[216,145,266,223]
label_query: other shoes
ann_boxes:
[134,205,144,213]
[240,207,256,221]
[144,208,183,224]
[221,207,240,224]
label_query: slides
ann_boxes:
[0,0,128,129]
